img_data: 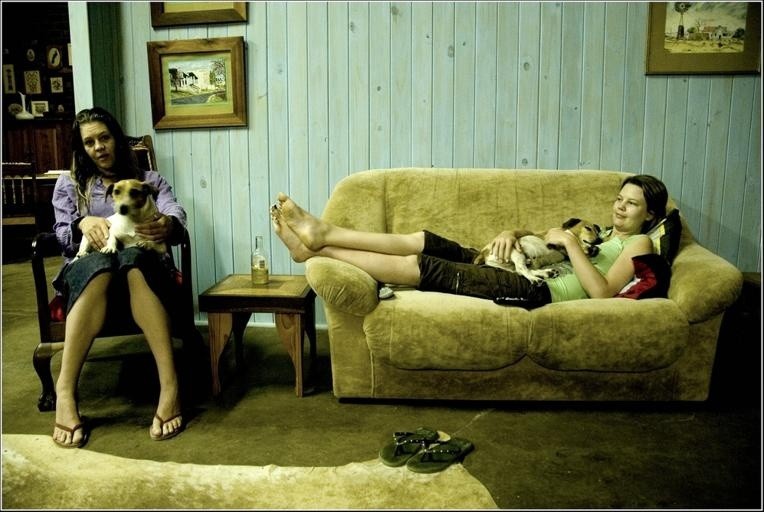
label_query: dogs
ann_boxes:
[473,217,603,288]
[68,179,171,265]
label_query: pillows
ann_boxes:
[614,206,684,305]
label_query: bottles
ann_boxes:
[250,236,270,285]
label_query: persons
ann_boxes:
[49,106,187,448]
[269,173,670,310]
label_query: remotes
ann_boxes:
[378,284,394,299]
[495,297,530,306]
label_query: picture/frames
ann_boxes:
[143,1,250,134]
[647,0,763,78]
[1,42,74,122]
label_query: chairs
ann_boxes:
[29,214,196,413]
[4,159,40,237]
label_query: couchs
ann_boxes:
[307,161,739,406]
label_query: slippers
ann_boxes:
[52,422,87,448]
[148,412,184,442]
[406,437,474,473]
[378,427,439,467]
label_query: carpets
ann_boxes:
[0,430,500,510]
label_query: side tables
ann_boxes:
[200,273,318,400]
